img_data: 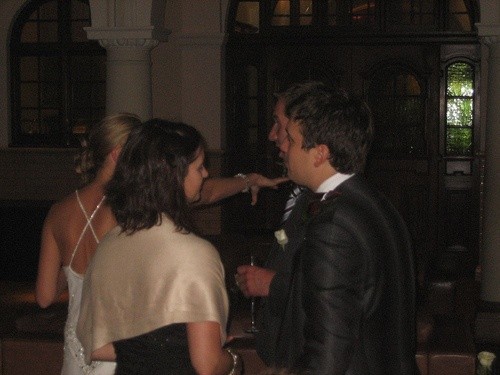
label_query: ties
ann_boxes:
[280,183,308,228]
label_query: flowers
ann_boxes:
[478,349,497,368]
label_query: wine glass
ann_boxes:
[240,255,261,334]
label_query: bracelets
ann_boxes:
[224,349,238,375]
[233,174,250,192]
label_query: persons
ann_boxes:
[258,89,422,374]
[77,115,237,375]
[32,112,291,375]
[235,81,335,375]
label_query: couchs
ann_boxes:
[0,267,477,374]
